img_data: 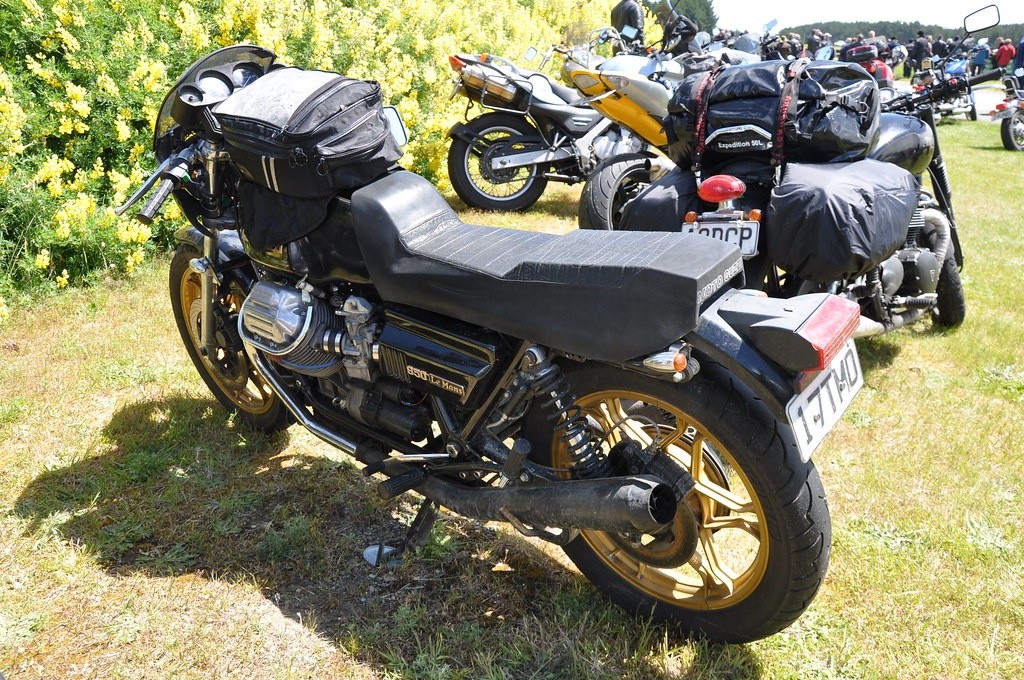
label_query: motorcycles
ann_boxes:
[991,64,1024,151]
[837,31,894,91]
[579,27,818,230]
[110,43,867,643]
[618,2,1002,333]
[445,9,751,213]
[909,36,991,128]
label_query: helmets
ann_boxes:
[560,53,607,89]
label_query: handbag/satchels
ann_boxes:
[763,158,923,285]
[616,164,701,232]
[210,64,406,200]
[660,54,882,173]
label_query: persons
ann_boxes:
[715,27,1024,96]
[610,0,645,56]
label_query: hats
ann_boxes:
[824,32,833,38]
[1003,38,1012,43]
[994,37,1004,44]
[890,36,897,40]
[916,30,925,36]
